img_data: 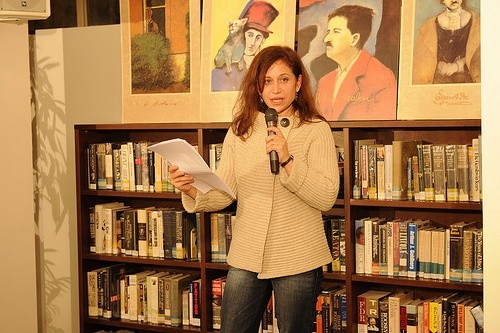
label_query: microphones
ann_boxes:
[265,108,279,173]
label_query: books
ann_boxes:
[358,290,484,333]
[353,137,482,202]
[213,276,347,333]
[87,142,198,193]
[211,213,346,272]
[355,216,484,284]
[88,265,200,327]
[89,202,201,261]
[209,144,344,199]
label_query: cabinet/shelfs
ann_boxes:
[74,119,483,333]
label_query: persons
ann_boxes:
[169,46,340,333]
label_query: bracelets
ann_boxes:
[281,155,293,167]
